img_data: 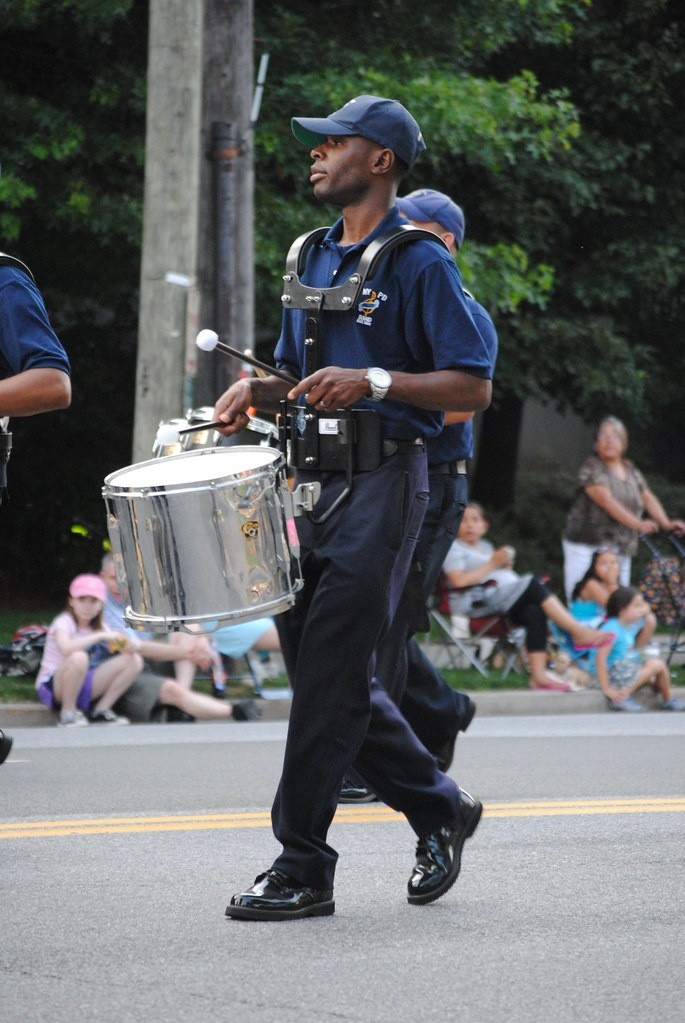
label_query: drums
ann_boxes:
[149,404,280,460]
[103,445,295,634]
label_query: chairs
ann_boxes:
[428,568,550,677]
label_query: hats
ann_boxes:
[69,573,107,603]
[290,94,427,170]
[394,188,466,251]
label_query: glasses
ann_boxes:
[593,545,621,556]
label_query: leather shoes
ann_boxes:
[338,781,377,803]
[431,693,477,775]
[225,870,336,921]
[407,785,483,906]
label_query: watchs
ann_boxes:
[363,367,393,404]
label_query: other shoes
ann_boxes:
[88,711,130,727]
[659,698,685,711]
[55,710,89,729]
[608,695,642,713]
[230,698,265,723]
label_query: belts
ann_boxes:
[378,435,424,459]
[428,458,468,475]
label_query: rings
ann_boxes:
[319,400,329,408]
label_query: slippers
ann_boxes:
[533,681,571,692]
[573,633,616,651]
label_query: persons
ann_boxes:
[97,552,264,723]
[34,573,145,729]
[210,93,501,921]
[212,616,282,660]
[440,501,618,691]
[561,415,685,603]
[569,546,657,650]
[587,587,685,713]
[0,252,76,764]
